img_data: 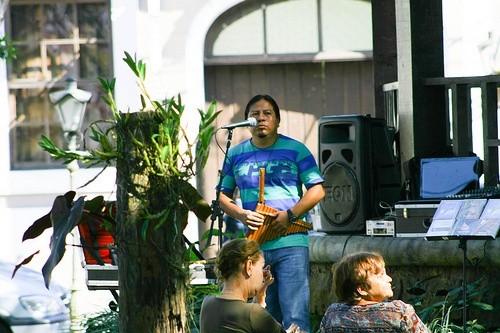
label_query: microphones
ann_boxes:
[220,117,257,129]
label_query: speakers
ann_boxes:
[317,113,402,233]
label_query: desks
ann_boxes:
[89,287,119,303]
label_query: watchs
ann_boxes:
[287,209,297,223]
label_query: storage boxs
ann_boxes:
[394,204,439,238]
[77,200,224,286]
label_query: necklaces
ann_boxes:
[251,141,274,175]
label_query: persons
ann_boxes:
[216,95,325,333]
[319,252,432,333]
[199,238,298,333]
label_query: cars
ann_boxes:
[0,260,72,333]
